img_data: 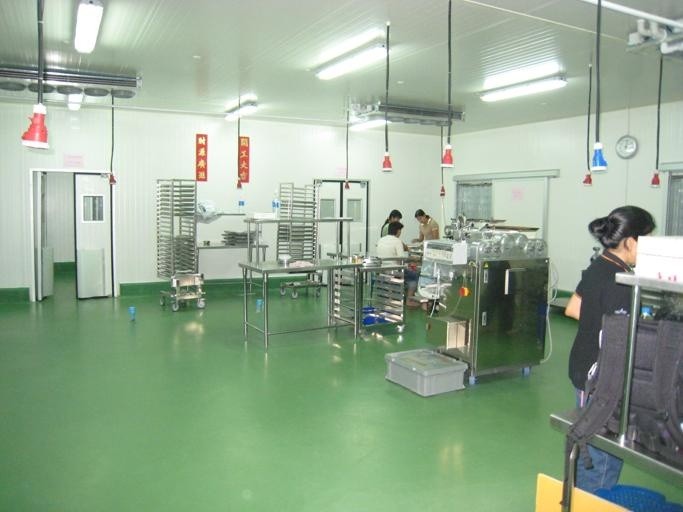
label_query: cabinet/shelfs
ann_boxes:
[549,272,683,512]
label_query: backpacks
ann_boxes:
[569,314,683,470]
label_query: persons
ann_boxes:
[375,221,421,307]
[381,209,408,251]
[565,205,657,495]
[411,209,440,243]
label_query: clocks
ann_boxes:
[615,135,638,160]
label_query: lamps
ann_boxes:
[75,2,104,54]
[478,74,567,103]
[225,102,255,122]
[315,39,387,82]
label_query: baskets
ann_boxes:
[593,485,681,512]
